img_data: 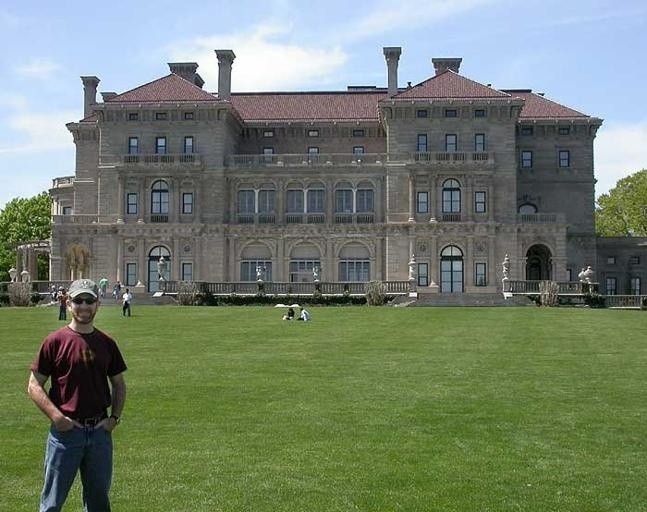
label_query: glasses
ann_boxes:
[71,297,97,304]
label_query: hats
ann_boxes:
[68,279,99,298]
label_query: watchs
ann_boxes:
[110,415,120,425]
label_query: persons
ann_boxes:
[113,280,121,301]
[50,284,69,321]
[282,307,295,322]
[122,288,133,318]
[296,307,310,322]
[26,278,128,512]
[98,276,109,299]
[257,277,265,295]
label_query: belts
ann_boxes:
[68,417,102,427]
